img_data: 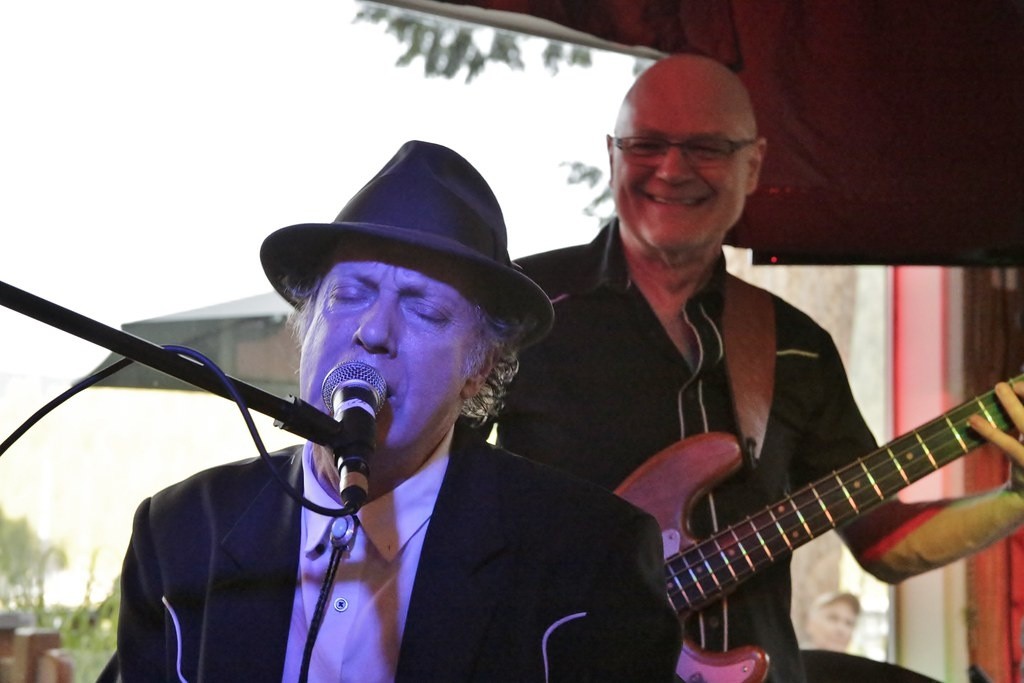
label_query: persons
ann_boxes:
[455,53,1024,683]
[119,141,685,683]
[806,591,859,651]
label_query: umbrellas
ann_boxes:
[69,290,304,401]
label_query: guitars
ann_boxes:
[615,374,1024,683]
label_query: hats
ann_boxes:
[259,141,555,352]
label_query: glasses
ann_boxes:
[611,136,756,170]
